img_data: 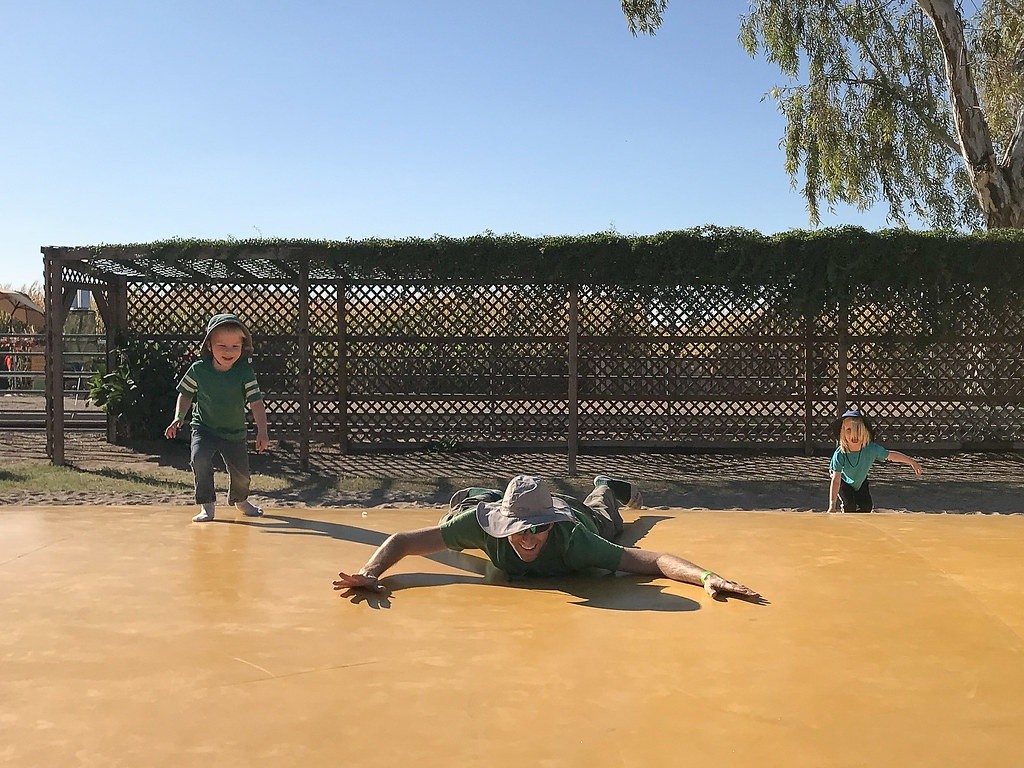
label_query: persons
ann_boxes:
[826,411,922,513]
[164,314,269,523]
[333,474,762,599]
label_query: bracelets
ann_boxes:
[698,569,714,586]
[174,411,186,420]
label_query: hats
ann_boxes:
[833,411,872,435]
[200,314,252,361]
[476,475,576,537]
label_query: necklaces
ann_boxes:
[843,444,864,468]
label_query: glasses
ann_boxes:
[516,524,550,535]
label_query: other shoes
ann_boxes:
[450,487,503,508]
[594,475,643,510]
